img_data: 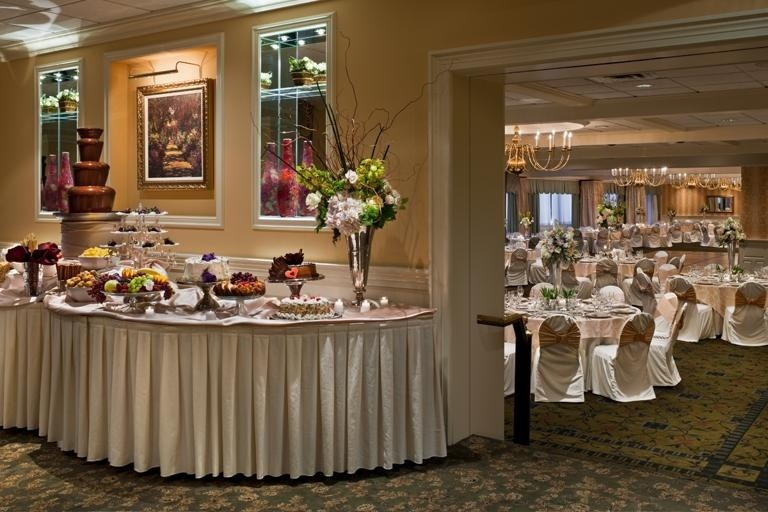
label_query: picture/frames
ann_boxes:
[136,78,213,192]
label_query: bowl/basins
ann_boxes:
[79,254,121,270]
[64,284,99,304]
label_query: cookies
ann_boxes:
[66,271,95,289]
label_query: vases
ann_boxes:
[277,138,298,217]
[298,141,317,216]
[261,83,270,89]
[42,106,56,113]
[347,226,374,307]
[59,101,77,112]
[314,75,327,83]
[292,72,314,85]
[261,142,279,215]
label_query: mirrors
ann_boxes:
[706,195,734,213]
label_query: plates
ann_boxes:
[100,207,181,253]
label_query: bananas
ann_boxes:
[136,268,160,276]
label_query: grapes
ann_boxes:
[230,271,262,293]
[86,273,175,302]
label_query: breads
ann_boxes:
[213,281,242,294]
[0,261,12,282]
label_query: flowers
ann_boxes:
[666,208,677,217]
[716,217,747,246]
[261,73,272,84]
[6,233,62,265]
[251,32,453,246]
[314,62,327,75]
[56,89,79,102]
[41,94,58,108]
[596,199,628,226]
[520,211,534,229]
[696,207,707,215]
[289,56,317,74]
[635,208,645,216]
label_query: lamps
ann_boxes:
[505,125,572,178]
[611,166,742,191]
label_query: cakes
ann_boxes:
[288,262,316,278]
[183,253,230,282]
[276,295,329,316]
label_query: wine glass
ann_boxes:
[504,283,638,321]
[579,248,650,265]
[680,262,768,285]
[214,292,264,317]
